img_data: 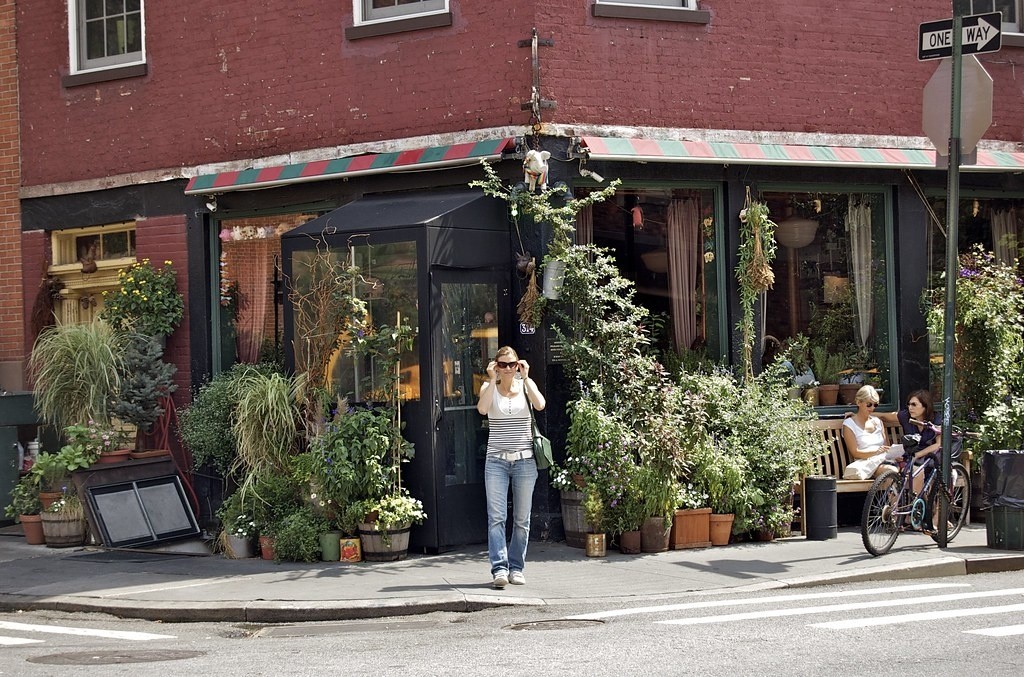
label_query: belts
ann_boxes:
[493,449,534,461]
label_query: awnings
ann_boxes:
[576,134,1024,173]
[181,134,524,199]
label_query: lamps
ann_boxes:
[772,197,820,249]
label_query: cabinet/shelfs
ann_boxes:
[0,391,67,520]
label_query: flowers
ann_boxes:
[564,454,588,474]
[231,513,256,540]
[310,492,336,531]
[48,485,78,513]
[549,438,637,531]
[957,241,1024,470]
[751,485,801,529]
[98,257,185,336]
[311,399,394,503]
[62,420,117,454]
[553,468,577,491]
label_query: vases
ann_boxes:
[228,535,254,558]
[572,474,586,487]
[560,490,594,549]
[319,530,343,561]
[970,471,986,523]
[758,529,774,540]
[41,511,87,548]
[97,448,131,463]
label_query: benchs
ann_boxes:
[779,419,973,537]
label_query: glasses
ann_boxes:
[860,400,878,407]
[497,361,518,368]
[905,403,922,407]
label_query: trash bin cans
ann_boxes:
[805,474,838,540]
[984,449,1024,552]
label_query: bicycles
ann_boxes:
[860,419,986,556]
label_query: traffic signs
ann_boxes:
[918,11,1002,62]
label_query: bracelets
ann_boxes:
[524,375,529,382]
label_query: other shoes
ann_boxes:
[901,522,923,533]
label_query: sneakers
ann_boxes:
[494,569,525,587]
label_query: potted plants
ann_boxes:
[468,156,623,300]
[336,495,428,562]
[580,342,878,557]
[111,337,171,460]
[30,443,90,509]
[4,474,46,544]
[246,472,296,560]
[153,333,166,348]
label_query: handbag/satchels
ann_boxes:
[842,451,888,480]
[523,382,553,469]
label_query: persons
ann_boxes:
[840,385,928,533]
[476,346,546,587]
[843,389,958,534]
